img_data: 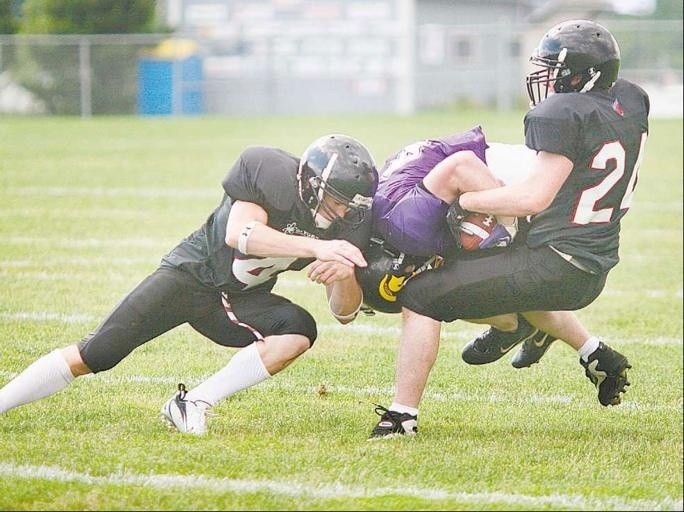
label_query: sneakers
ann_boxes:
[367,411,419,441]
[161,390,207,435]
[579,342,632,407]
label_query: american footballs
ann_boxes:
[460,211,497,251]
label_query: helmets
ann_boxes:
[524,20,620,110]
[296,134,380,240]
[353,243,442,315]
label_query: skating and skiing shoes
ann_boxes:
[460,314,559,368]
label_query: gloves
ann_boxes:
[478,216,519,250]
[445,195,469,249]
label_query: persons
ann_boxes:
[350,128,564,368]
[368,18,651,440]
[1,133,378,443]
